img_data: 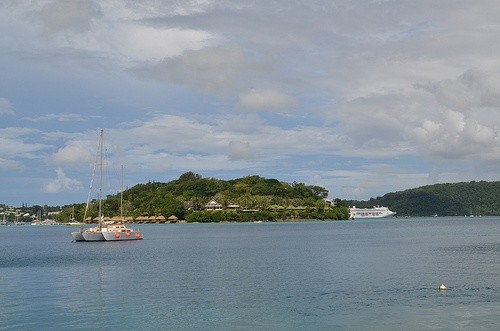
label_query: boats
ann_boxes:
[323,199,398,219]
[40,219,57,225]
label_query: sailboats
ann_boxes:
[31,210,41,225]
[68,206,80,223]
[70,130,143,242]
[0,204,8,225]
[14,211,24,225]
